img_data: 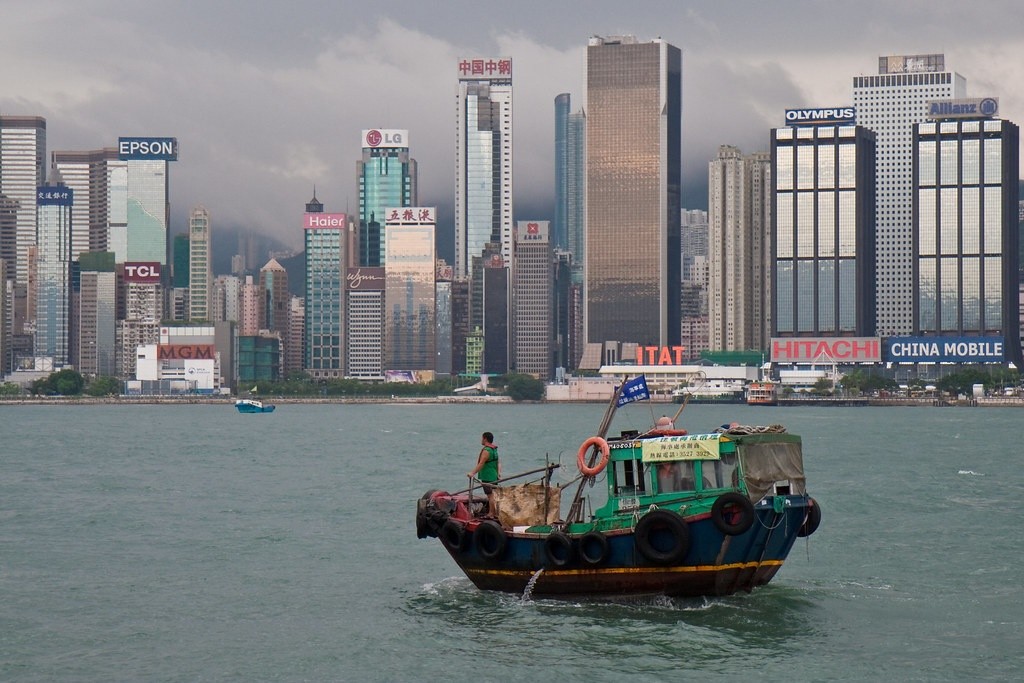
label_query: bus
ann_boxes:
[760,361,837,386]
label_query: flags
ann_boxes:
[614,376,648,407]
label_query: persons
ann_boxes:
[468,431,501,518]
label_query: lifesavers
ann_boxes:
[537,528,615,572]
[794,496,824,541]
[631,504,699,570]
[646,427,689,439]
[575,434,612,479]
[708,487,758,539]
[413,486,512,564]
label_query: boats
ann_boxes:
[415,378,822,604]
[747,381,778,405]
[234,399,276,414]
